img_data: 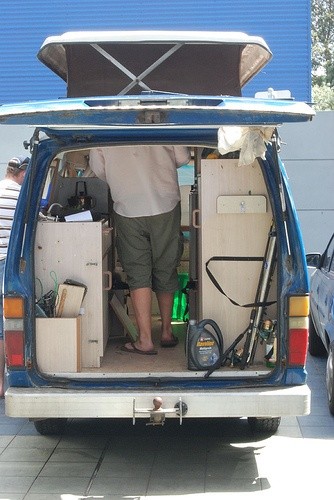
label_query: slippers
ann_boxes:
[120,341,159,355]
[160,334,179,348]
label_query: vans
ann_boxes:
[0,29,315,438]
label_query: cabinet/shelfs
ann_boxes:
[33,213,114,368]
[193,157,277,364]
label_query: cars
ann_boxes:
[305,231,333,418]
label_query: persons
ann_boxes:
[0,154,31,399]
[89,145,191,356]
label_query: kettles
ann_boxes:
[68,181,91,211]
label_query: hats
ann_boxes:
[8,155,30,170]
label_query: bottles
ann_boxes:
[265,319,279,368]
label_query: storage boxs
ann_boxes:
[36,318,80,372]
